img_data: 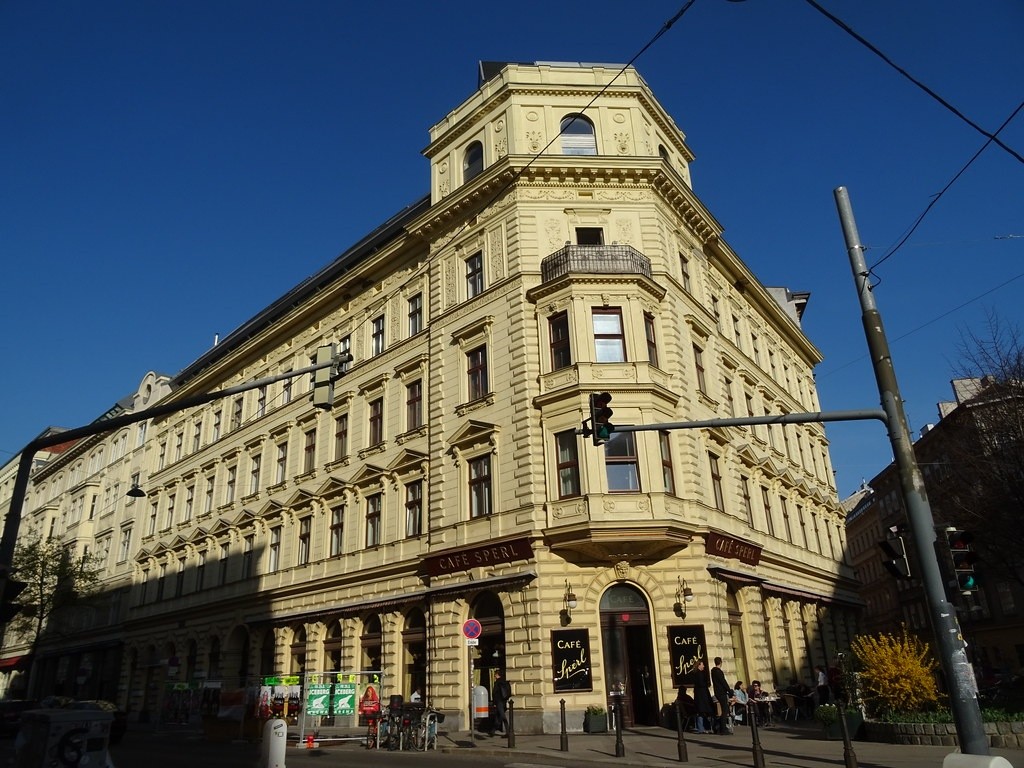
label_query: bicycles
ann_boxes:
[366,703,432,751]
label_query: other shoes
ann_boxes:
[501,734,509,738]
[487,729,495,735]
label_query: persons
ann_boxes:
[492,670,511,738]
[973,656,1004,702]
[712,681,770,726]
[693,660,714,733]
[711,657,734,735]
[786,665,830,720]
[411,686,426,702]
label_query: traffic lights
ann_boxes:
[947,527,979,592]
[876,537,913,578]
[590,390,614,447]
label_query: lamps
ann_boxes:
[566,583,577,608]
[682,578,693,602]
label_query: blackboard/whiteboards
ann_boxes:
[550,628,593,694]
[666,624,711,689]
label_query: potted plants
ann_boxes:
[585,704,608,736]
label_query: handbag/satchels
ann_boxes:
[734,713,742,721]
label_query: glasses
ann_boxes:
[752,685,758,687]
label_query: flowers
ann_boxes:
[816,703,855,724]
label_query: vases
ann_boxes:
[824,717,862,740]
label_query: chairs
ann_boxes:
[711,702,733,734]
[680,704,696,732]
[782,694,804,721]
